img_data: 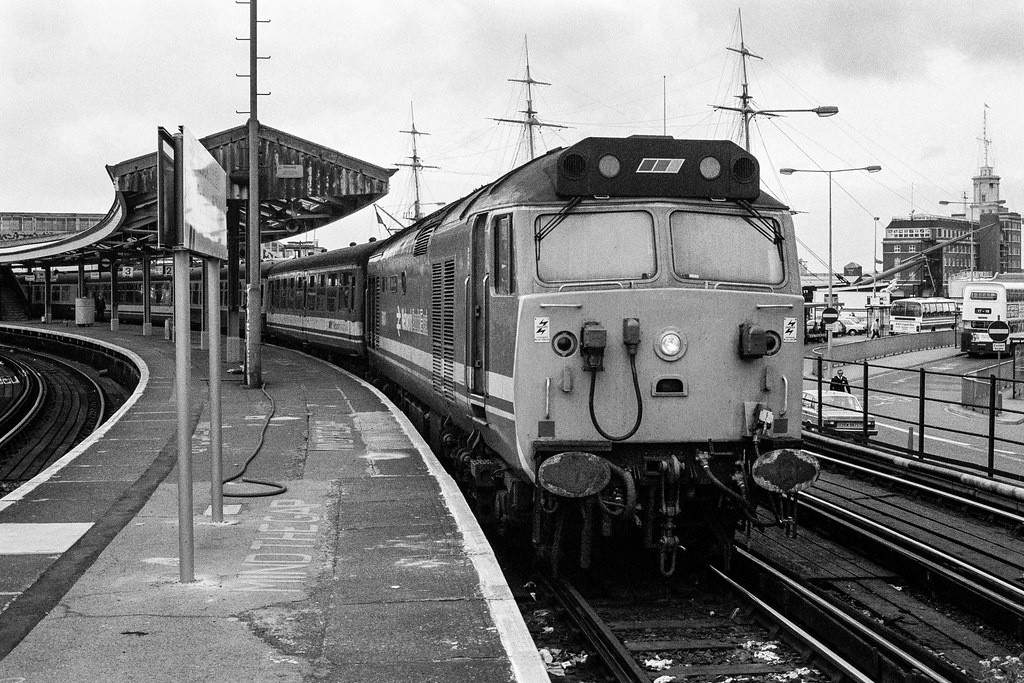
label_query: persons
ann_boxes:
[828,369,852,395]
[848,313,855,316]
[813,319,826,343]
[871,318,883,340]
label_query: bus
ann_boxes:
[960,280,1024,359]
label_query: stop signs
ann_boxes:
[988,320,1010,343]
[823,308,838,324]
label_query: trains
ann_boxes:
[21,135,818,576]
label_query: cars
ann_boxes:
[838,315,867,336]
[806,320,822,332]
[801,388,880,443]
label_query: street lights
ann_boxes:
[779,165,882,386]
[939,200,1006,279]
[873,216,879,297]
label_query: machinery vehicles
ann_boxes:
[890,297,960,335]
